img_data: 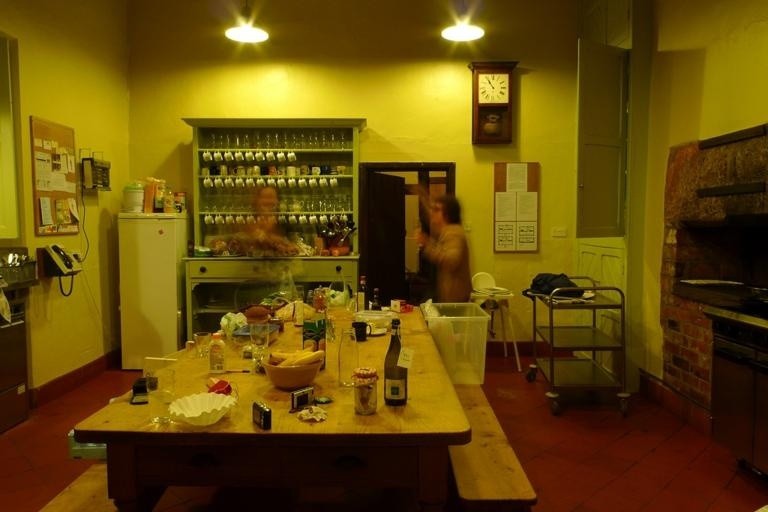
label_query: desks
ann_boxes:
[463,286,525,375]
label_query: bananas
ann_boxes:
[269,347,324,367]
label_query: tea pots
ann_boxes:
[315,219,356,256]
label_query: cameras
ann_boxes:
[290,385,314,411]
[252,400,272,430]
[133,376,158,395]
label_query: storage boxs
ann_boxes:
[416,298,493,389]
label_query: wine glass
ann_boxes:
[209,128,347,149]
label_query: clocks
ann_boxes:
[465,56,522,147]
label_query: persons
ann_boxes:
[229,186,298,311]
[411,183,472,304]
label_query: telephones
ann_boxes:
[42,245,83,276]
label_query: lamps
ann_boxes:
[437,0,485,43]
[222,1,269,45]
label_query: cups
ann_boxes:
[144,367,176,425]
[199,151,352,226]
[249,322,269,357]
[194,331,210,361]
[351,320,372,341]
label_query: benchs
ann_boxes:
[438,376,540,512]
[33,453,123,512]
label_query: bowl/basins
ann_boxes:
[235,323,280,345]
[261,356,327,391]
[167,392,240,425]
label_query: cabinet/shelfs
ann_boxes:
[568,242,643,395]
[574,35,650,250]
[574,0,651,51]
[181,113,371,345]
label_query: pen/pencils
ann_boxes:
[223,369,250,373]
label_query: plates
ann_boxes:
[359,322,388,336]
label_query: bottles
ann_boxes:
[383,318,408,407]
[337,324,359,388]
[327,265,351,318]
[209,332,226,372]
[292,287,306,326]
[277,270,297,303]
[357,275,369,311]
[154,187,176,212]
[324,312,336,342]
[292,283,383,318]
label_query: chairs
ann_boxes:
[468,271,500,337]
